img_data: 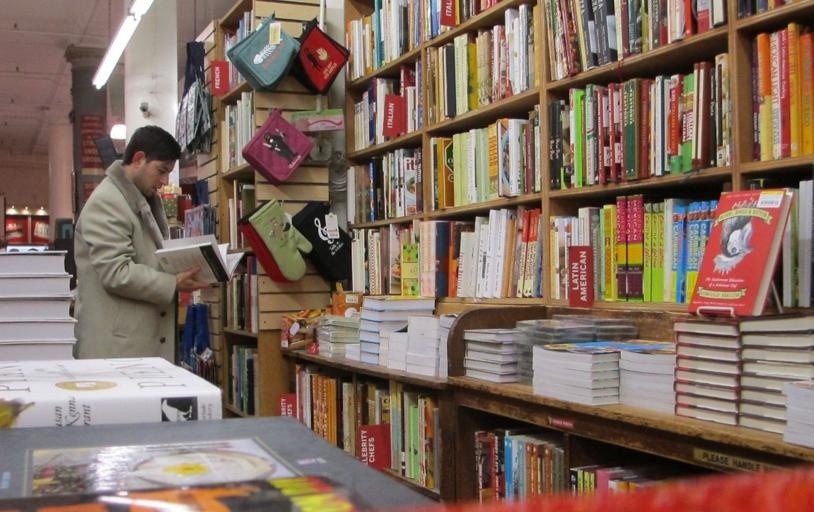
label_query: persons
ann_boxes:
[75,125,209,360]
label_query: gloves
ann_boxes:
[248,198,313,281]
[237,202,300,284]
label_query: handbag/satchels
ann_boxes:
[226,11,299,92]
[244,106,313,185]
[293,201,354,283]
[293,17,351,95]
[180,303,217,385]
[174,42,213,156]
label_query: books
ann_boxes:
[155,232,245,289]
[0,357,373,512]
[1,252,78,361]
[347,1,421,298]
[544,0,814,317]
[317,294,813,503]
[423,1,543,297]
[164,10,254,416]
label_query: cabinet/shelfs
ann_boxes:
[338,1,814,316]
[215,0,332,420]
[279,343,454,511]
[445,299,814,512]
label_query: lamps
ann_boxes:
[89,1,141,91]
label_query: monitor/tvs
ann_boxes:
[92,134,124,171]
